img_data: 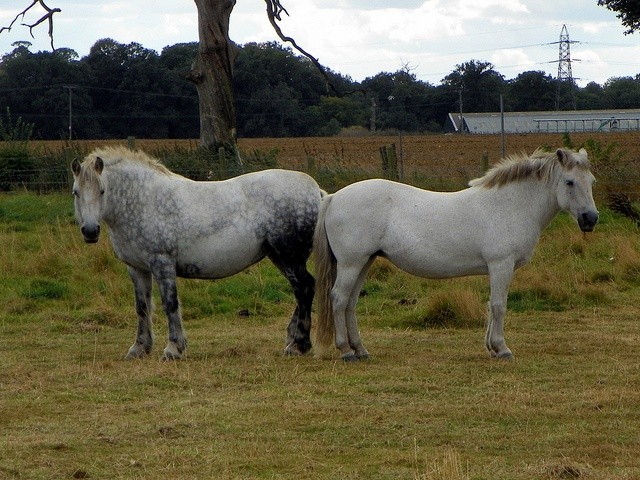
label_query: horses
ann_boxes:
[70,145,328,359]
[311,147,599,360]
